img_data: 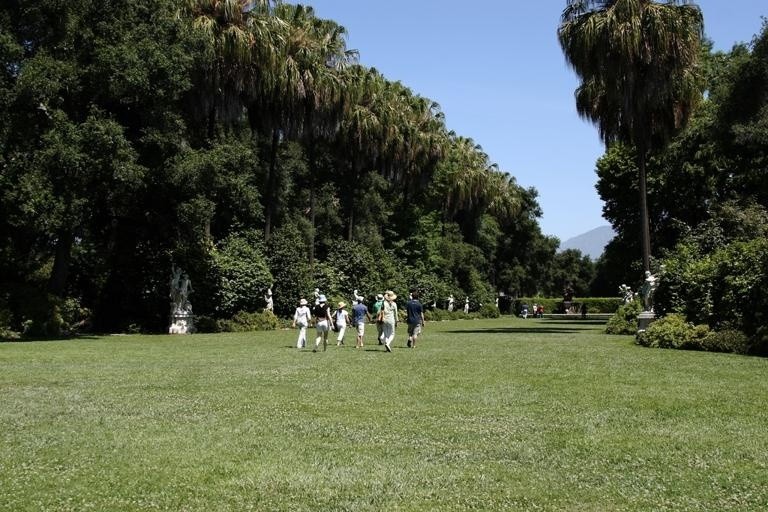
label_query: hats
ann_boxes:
[336,302,348,310]
[383,291,398,302]
[375,294,384,302]
[356,295,365,301]
[299,299,308,306]
[318,294,328,302]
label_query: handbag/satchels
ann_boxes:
[378,304,384,323]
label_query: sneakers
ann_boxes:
[296,337,418,353]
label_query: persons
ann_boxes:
[446,294,455,311]
[354,289,365,302]
[619,282,634,304]
[179,274,195,300]
[312,293,335,353]
[537,304,544,318]
[352,298,374,349]
[332,301,351,346]
[168,263,186,302]
[292,298,313,350]
[376,290,399,352]
[532,303,538,318]
[312,287,321,310]
[640,269,659,313]
[464,295,470,314]
[371,293,385,345]
[406,292,425,348]
[579,303,587,319]
[521,302,529,319]
[264,282,276,312]
